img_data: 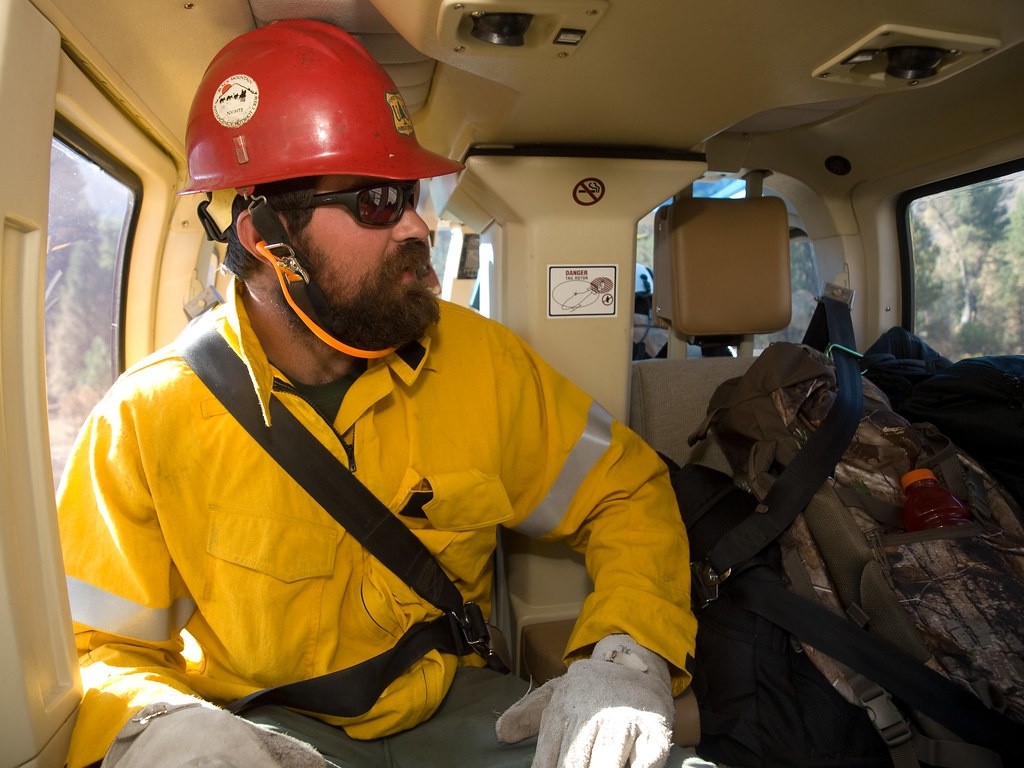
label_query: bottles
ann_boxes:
[901,468,973,533]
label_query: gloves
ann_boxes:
[100,702,328,768]
[494,634,675,768]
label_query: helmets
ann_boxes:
[175,19,466,196]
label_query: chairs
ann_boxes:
[624,190,795,463]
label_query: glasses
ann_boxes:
[278,181,421,228]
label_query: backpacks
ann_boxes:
[687,341,1024,768]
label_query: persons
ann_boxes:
[52,19,717,768]
